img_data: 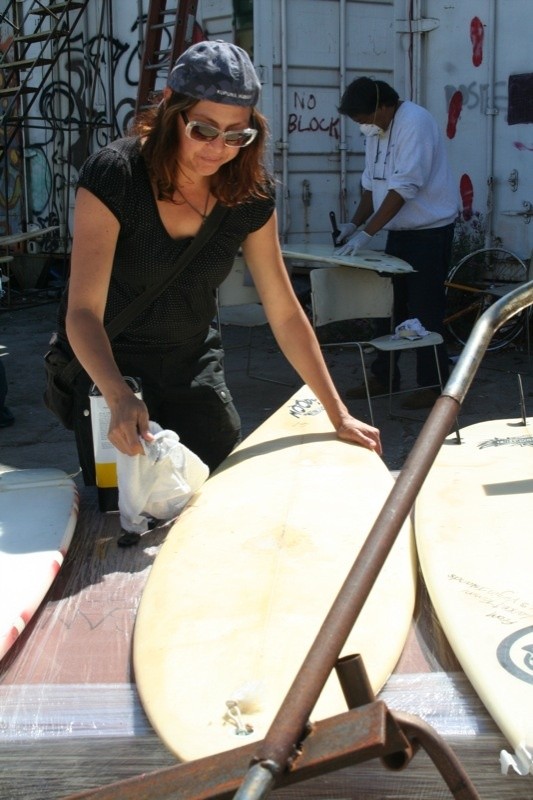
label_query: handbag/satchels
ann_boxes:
[42,349,80,428]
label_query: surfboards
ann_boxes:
[132,383,417,763]
[239,243,414,274]
[414,374,533,777]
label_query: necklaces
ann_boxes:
[176,186,211,223]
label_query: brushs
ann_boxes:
[328,210,345,248]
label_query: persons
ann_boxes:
[335,76,462,411]
[42,40,383,513]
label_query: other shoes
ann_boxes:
[401,388,438,408]
[0,406,14,427]
[346,376,394,399]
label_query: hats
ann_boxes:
[167,39,260,106]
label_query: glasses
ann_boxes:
[179,108,258,148]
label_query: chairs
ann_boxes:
[309,268,462,447]
[213,254,314,388]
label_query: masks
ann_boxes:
[359,123,382,138]
